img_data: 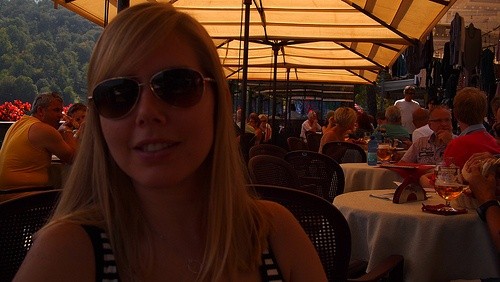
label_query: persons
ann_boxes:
[236,85,500,183]
[12,3,328,282]
[462,152,500,254]
[0,92,88,202]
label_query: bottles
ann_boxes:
[367,136,379,165]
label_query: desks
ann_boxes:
[378,143,408,161]
[48,161,72,187]
[331,188,500,282]
[329,163,435,192]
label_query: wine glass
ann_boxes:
[374,145,393,165]
[434,171,463,211]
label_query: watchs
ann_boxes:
[476,199,500,222]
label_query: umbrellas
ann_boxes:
[51,0,457,160]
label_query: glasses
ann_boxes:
[248,119,255,123]
[430,117,452,125]
[84,68,215,121]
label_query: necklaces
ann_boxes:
[144,210,208,275]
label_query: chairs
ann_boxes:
[307,133,322,151]
[287,137,308,151]
[284,150,346,203]
[248,155,309,192]
[245,185,405,282]
[323,142,367,164]
[0,188,63,282]
[248,144,287,158]
